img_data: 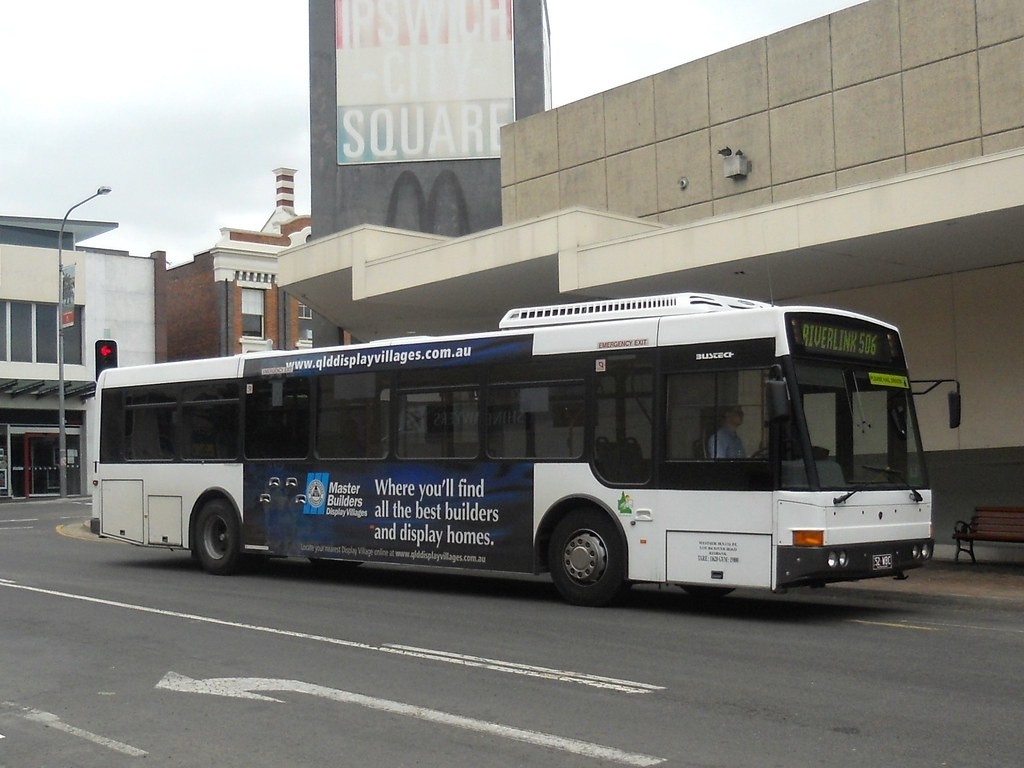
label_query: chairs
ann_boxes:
[595,435,650,483]
[691,423,718,460]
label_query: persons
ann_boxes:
[708,406,747,459]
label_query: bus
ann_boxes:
[90,291,963,608]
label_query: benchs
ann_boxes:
[953,507,1024,563]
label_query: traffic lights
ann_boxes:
[95,340,117,385]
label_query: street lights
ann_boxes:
[59,185,115,499]
